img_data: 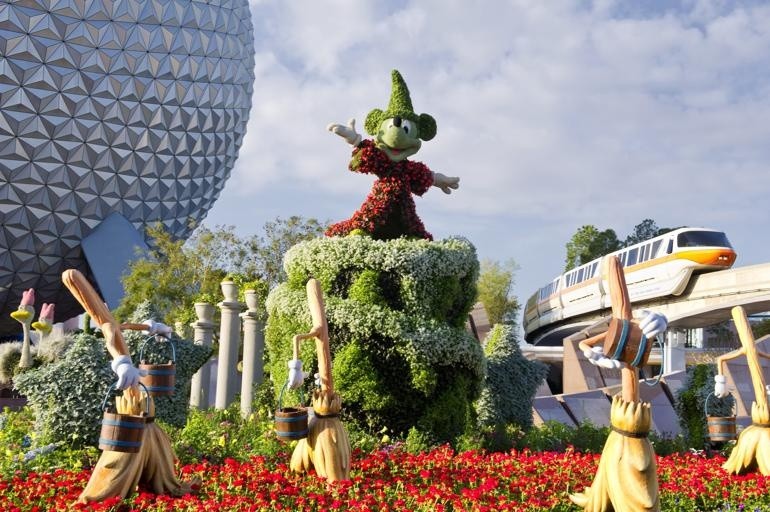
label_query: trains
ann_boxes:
[522,228,738,343]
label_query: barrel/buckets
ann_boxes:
[704,390,739,441]
[137,336,177,396]
[99,382,150,452]
[276,383,309,437]
[602,320,664,389]
